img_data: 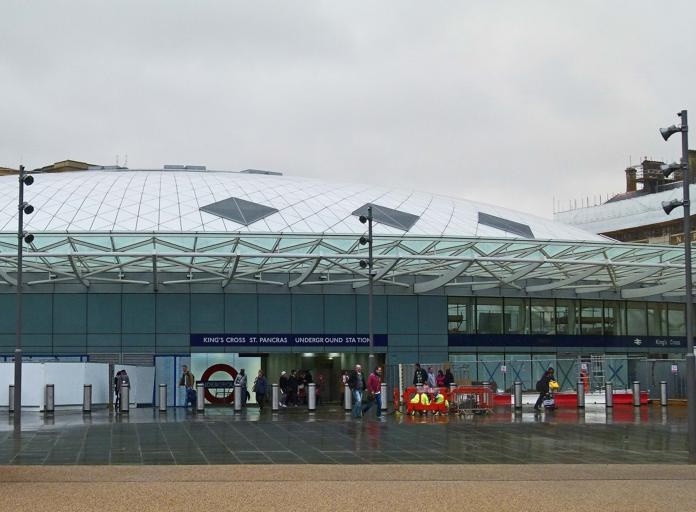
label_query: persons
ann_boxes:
[343,364,384,419]
[413,364,454,387]
[534,367,553,411]
[279,369,325,408]
[234,369,247,407]
[177,365,194,410]
[252,370,268,413]
[114,370,131,409]
